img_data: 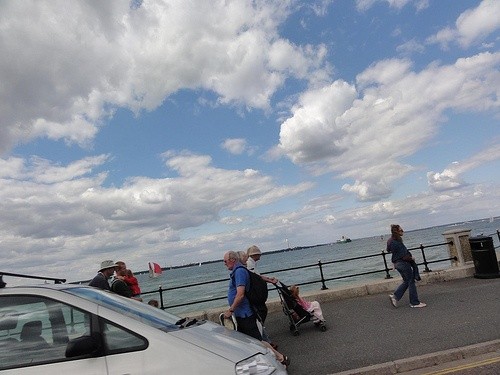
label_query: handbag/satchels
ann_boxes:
[411,261,422,281]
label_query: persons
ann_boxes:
[88,259,143,303]
[234,245,279,351]
[223,249,291,372]
[287,284,326,327]
[385,223,427,308]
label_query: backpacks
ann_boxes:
[230,266,268,306]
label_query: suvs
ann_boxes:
[0,272,288,375]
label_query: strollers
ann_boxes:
[272,278,327,334]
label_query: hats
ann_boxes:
[96,260,119,273]
[247,245,262,255]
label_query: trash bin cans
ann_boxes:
[469,235,500,279]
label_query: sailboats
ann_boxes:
[147,262,162,280]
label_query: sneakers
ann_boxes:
[388,294,400,308]
[410,302,427,308]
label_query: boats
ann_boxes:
[335,235,351,243]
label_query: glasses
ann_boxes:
[399,229,403,232]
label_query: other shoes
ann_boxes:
[270,343,278,349]
[281,353,291,365]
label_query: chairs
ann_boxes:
[0,317,57,353]
